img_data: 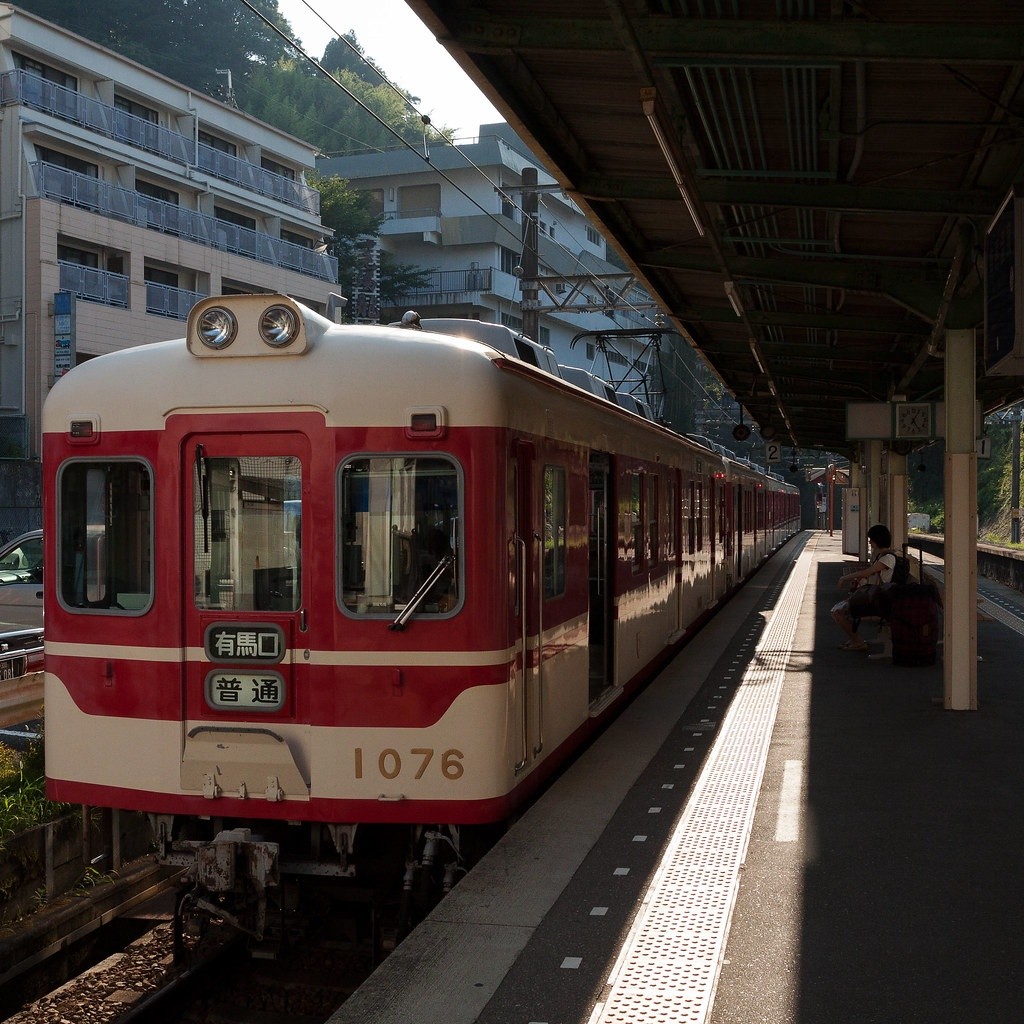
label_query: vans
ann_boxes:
[0,525,106,679]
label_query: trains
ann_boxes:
[38,285,803,968]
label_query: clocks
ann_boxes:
[892,402,936,441]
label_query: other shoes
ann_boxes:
[839,642,867,651]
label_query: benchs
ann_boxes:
[843,550,993,663]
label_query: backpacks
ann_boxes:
[872,550,910,585]
[850,584,886,634]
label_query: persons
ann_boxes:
[830,525,897,652]
